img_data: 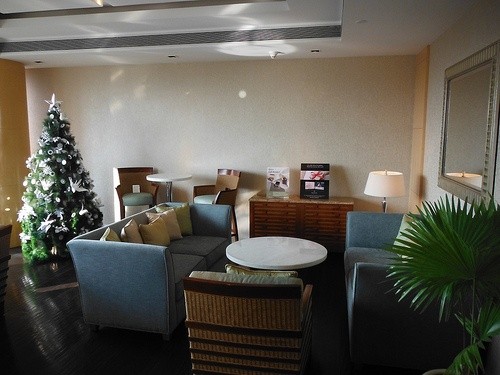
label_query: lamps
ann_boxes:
[447,171,483,188]
[363,169,406,213]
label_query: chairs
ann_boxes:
[113,167,161,222]
[181,270,314,375]
[191,168,241,241]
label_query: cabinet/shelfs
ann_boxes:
[249,191,353,248]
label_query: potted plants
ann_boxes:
[378,191,500,375]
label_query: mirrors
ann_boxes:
[436,40,500,208]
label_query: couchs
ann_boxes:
[67,200,232,341]
[344,210,475,375]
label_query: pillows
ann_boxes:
[155,203,194,235]
[225,264,299,278]
[99,226,120,243]
[139,216,170,246]
[145,209,183,240]
[391,214,423,265]
[120,217,144,244]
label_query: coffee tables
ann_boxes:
[226,236,328,270]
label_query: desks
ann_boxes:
[145,173,192,201]
